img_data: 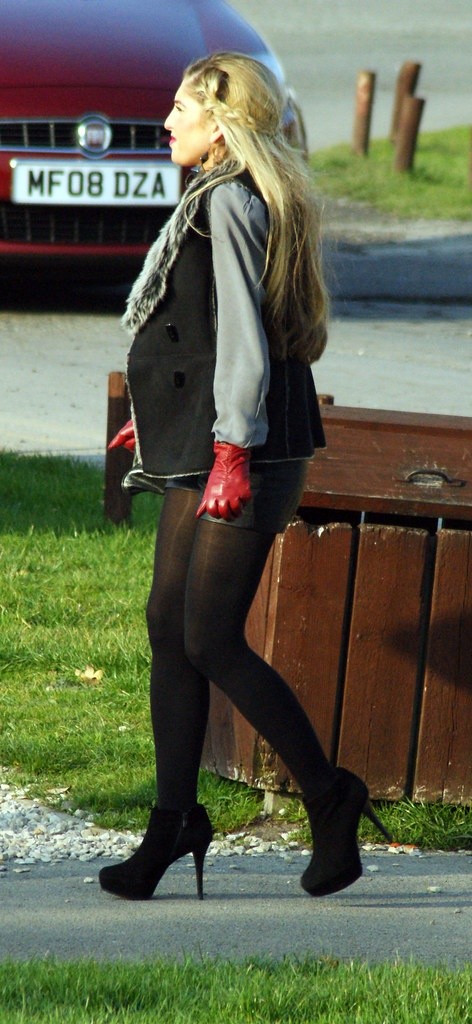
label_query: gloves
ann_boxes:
[195,442,252,522]
[107,419,137,454]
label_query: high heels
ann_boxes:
[98,804,213,901]
[301,767,392,896]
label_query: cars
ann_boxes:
[0,0,306,306]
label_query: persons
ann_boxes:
[98,53,392,902]
